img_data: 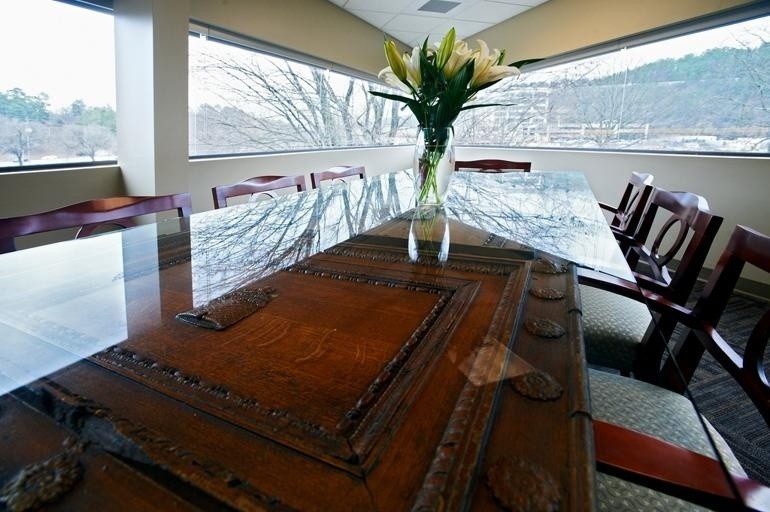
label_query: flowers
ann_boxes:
[367,28,519,203]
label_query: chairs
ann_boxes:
[311,165,368,191]
[454,159,532,172]
[578,186,725,385]
[211,172,309,209]
[579,222,769,511]
[0,192,192,255]
[595,171,655,256]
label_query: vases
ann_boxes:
[412,128,456,209]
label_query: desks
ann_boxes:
[0,171,754,509]
